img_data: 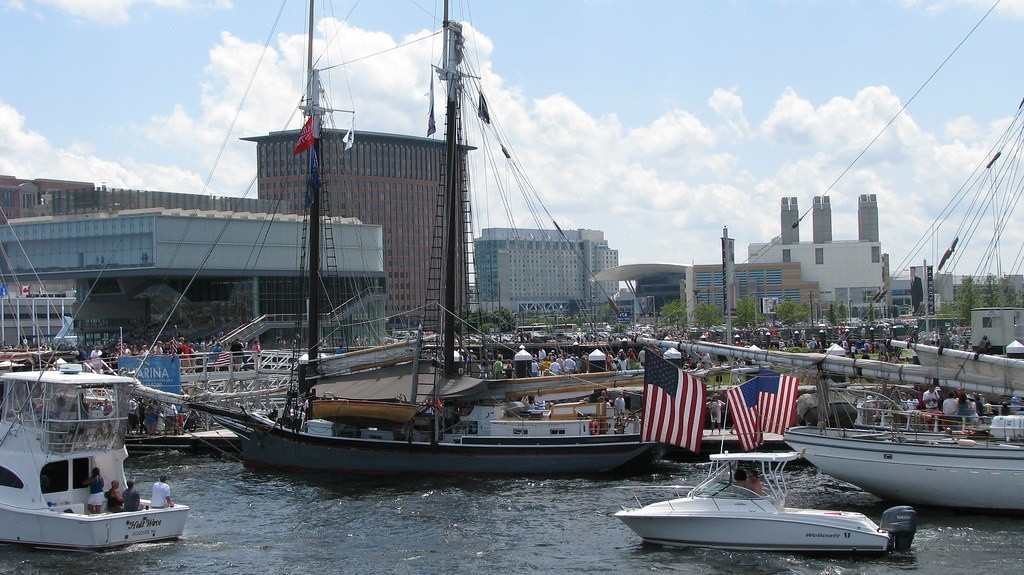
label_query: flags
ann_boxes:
[639,347,707,458]
[343,114,354,150]
[478,88,490,125]
[171,325,178,361]
[724,364,801,452]
[426,65,436,137]
[292,114,323,212]
[115,329,121,357]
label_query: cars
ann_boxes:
[388,329,675,341]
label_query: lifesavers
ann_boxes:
[591,421,600,435]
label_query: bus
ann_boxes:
[516,323,577,337]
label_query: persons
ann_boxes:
[35,391,112,423]
[290,392,309,429]
[769,299,778,313]
[273,333,368,351]
[126,393,185,434]
[151,473,174,509]
[104,480,126,513]
[910,267,923,315]
[122,480,140,511]
[466,320,1021,435]
[82,467,104,514]
[0,332,263,372]
[95,255,104,266]
[141,251,149,264]
[733,467,762,496]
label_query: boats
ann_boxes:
[127,0,692,485]
[0,359,191,550]
[612,448,918,553]
[630,331,1024,514]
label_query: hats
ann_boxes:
[558,354,562,357]
[946,391,956,397]
[967,394,975,399]
[551,350,555,353]
[934,387,941,391]
[711,393,721,398]
[601,390,605,393]
